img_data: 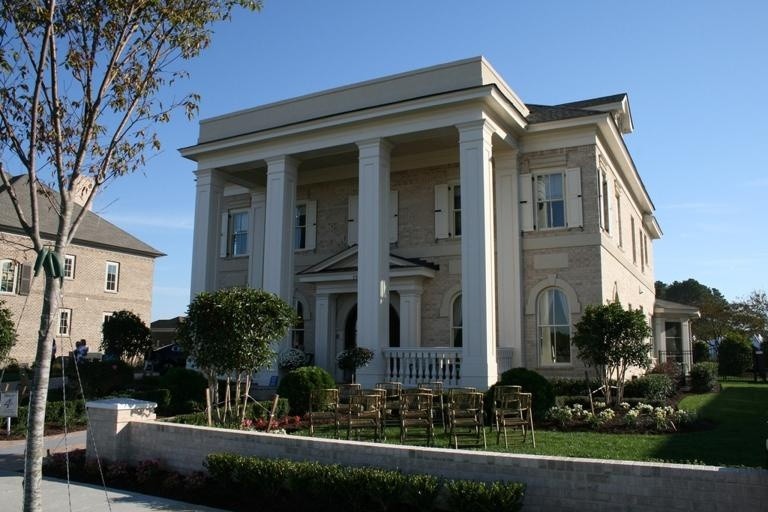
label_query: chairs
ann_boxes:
[309,381,536,450]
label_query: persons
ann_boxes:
[74,341,83,360]
[81,339,88,359]
[52,339,56,360]
